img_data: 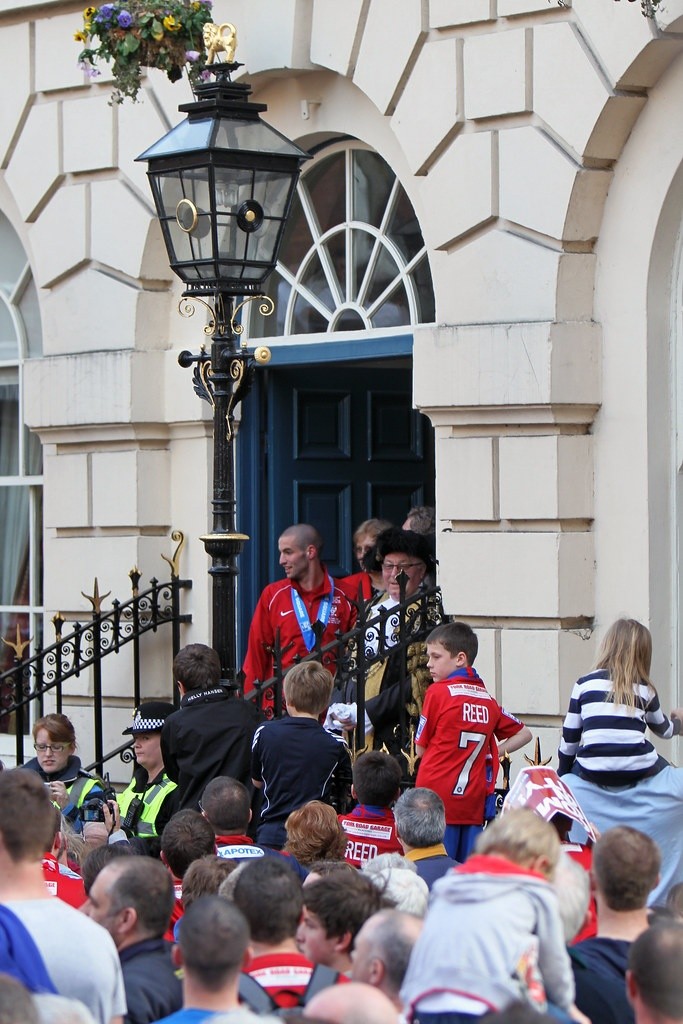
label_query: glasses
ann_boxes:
[381,563,423,572]
[34,741,72,752]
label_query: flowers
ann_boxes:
[73,0,215,109]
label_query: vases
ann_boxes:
[137,32,188,69]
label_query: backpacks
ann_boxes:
[238,963,339,1024]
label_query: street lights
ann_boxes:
[137,42,310,690]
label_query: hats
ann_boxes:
[122,702,174,735]
[376,530,435,572]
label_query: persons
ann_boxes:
[236,523,357,725]
[155,643,265,843]
[341,519,396,605]
[248,661,353,851]
[19,714,117,834]
[402,506,436,596]
[414,622,532,865]
[0,760,683,1024]
[116,702,184,839]
[556,619,683,845]
[337,751,406,869]
[323,529,449,796]
